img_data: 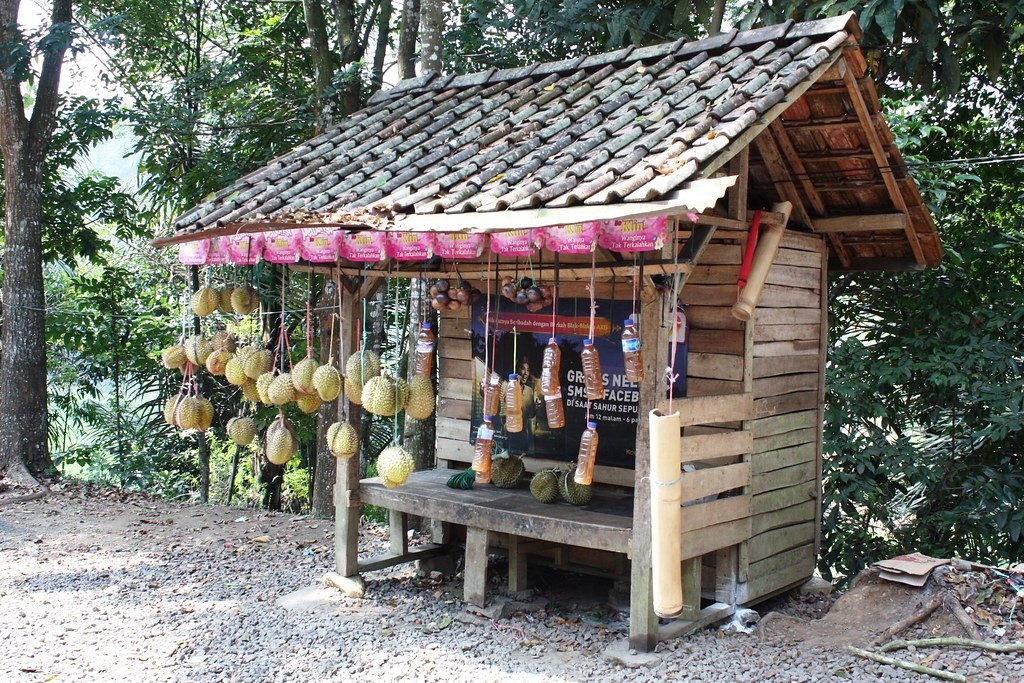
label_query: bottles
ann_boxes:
[574,422,598,485]
[506,374,522,432]
[472,415,494,471]
[416,322,434,378]
[581,339,604,400]
[475,455,491,483]
[541,338,561,395]
[544,383,565,429]
[485,374,500,416]
[621,319,643,382]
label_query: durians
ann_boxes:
[491,453,526,488]
[163,332,435,488]
[188,284,259,317]
[530,465,591,505]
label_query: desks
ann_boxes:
[332,468,702,652]
